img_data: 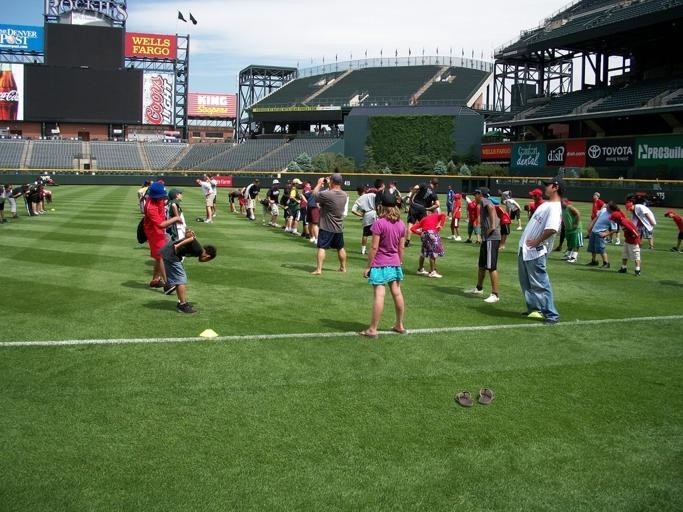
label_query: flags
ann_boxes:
[190,13,198,25]
[178,11,187,22]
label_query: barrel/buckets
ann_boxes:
[392,326,406,334]
[479,387,495,404]
[455,391,473,406]
[360,329,378,339]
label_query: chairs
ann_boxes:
[262,222,318,245]
[560,255,577,263]
[233,211,255,221]
[448,235,481,245]
[28,209,47,217]
[599,261,610,269]
[516,226,523,231]
[634,270,641,277]
[605,236,620,245]
[203,214,216,223]
[520,310,559,325]
[464,288,483,294]
[429,270,443,277]
[417,268,429,274]
[484,294,500,303]
[586,258,599,267]
[618,267,627,273]
[667,248,678,252]
[649,244,654,249]
[150,279,197,314]
[0,214,19,225]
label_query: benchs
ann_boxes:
[190,13,198,25]
[178,11,187,22]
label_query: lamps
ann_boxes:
[482,0,682,122]
[244,60,492,111]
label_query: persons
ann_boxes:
[463,187,502,303]
[196,175,219,223]
[355,190,408,334]
[138,177,187,295]
[1,174,55,224]
[516,174,567,321]
[228,174,448,278]
[159,237,216,313]
[445,175,682,276]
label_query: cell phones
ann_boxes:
[323,179,327,183]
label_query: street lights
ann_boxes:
[137,220,147,245]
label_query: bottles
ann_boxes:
[453,194,463,199]
[168,189,182,199]
[528,189,543,198]
[149,182,167,198]
[543,176,565,190]
[471,188,489,197]
[292,178,303,185]
[625,202,632,210]
[273,180,280,184]
[382,195,397,207]
[592,191,601,197]
[664,209,675,217]
[329,174,342,184]
